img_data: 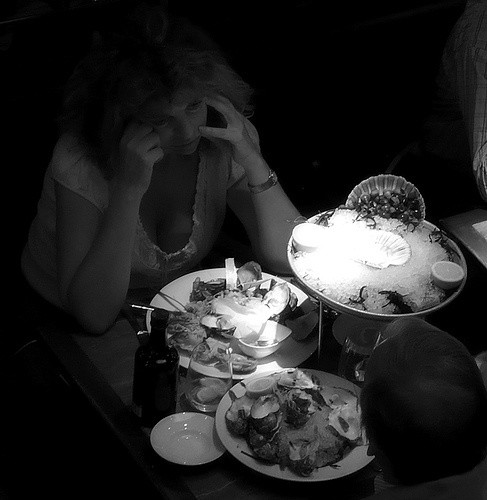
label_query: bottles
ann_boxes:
[131,310,180,428]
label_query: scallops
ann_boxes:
[224,369,363,477]
[166,262,320,374]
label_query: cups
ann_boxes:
[183,339,233,412]
[338,330,379,382]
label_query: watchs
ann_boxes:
[246,169,278,193]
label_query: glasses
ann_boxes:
[355,359,367,381]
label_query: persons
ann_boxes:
[441,0,487,202]
[357,318,487,499]
[24,28,307,333]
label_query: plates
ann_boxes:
[147,267,320,379]
[216,368,375,481]
[151,413,224,465]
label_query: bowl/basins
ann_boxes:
[293,223,326,252]
[432,261,464,289]
[232,319,292,359]
